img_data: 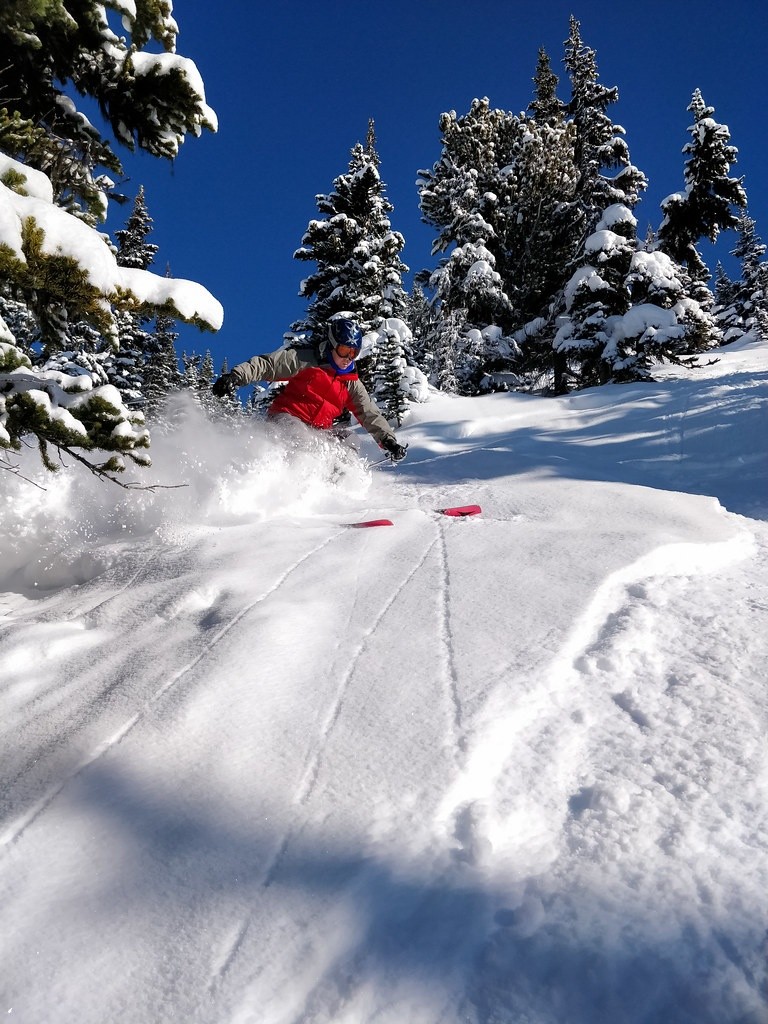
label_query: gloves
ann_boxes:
[384,439,408,462]
[213,371,243,398]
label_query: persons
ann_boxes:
[212,310,406,463]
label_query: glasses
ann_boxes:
[329,327,360,360]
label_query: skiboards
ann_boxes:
[338,502,483,529]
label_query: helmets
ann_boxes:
[328,319,363,351]
